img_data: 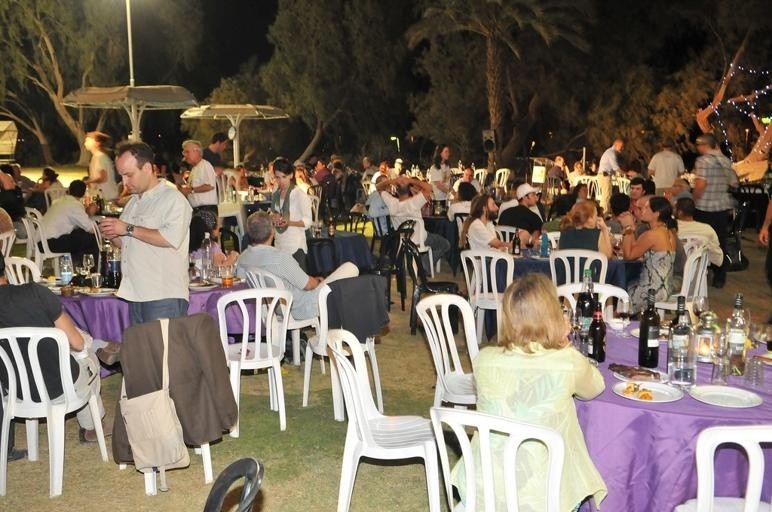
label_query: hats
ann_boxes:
[516,183,539,200]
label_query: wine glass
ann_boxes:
[611,239,621,259]
[83,254,95,278]
[91,273,104,292]
[525,239,535,254]
[568,308,590,357]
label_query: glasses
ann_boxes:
[181,148,197,155]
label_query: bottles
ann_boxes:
[576,269,748,387]
[434,201,442,218]
[60,255,74,286]
[254,184,259,204]
[327,207,336,240]
[541,228,548,256]
[100,237,122,288]
[513,224,520,254]
[201,231,214,282]
[96,189,104,216]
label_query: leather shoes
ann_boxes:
[9,447,31,461]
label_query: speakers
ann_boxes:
[481,128,497,153]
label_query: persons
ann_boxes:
[546,151,599,185]
[679,160,696,186]
[267,161,312,269]
[600,135,627,216]
[0,169,28,239]
[367,174,400,258]
[427,143,459,215]
[190,210,238,269]
[82,129,120,201]
[377,177,450,274]
[295,149,373,214]
[557,200,626,295]
[368,161,389,196]
[542,196,573,235]
[647,137,685,195]
[37,168,67,202]
[10,164,47,215]
[710,135,749,271]
[758,144,772,351]
[238,212,346,358]
[617,197,674,310]
[675,134,725,299]
[0,252,122,445]
[155,155,193,203]
[443,271,606,512]
[675,197,720,251]
[201,132,229,178]
[35,179,106,272]
[560,185,602,218]
[180,140,218,217]
[460,194,514,253]
[453,166,481,193]
[606,191,635,235]
[497,182,543,248]
[632,191,688,292]
[100,140,192,328]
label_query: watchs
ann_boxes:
[191,187,194,194]
[125,224,134,237]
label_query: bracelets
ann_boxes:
[624,226,633,231]
[317,278,322,285]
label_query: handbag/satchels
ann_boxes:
[119,388,191,474]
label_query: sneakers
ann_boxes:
[82,420,116,442]
[96,341,128,366]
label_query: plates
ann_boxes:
[211,278,246,286]
[79,288,116,297]
[689,385,764,408]
[753,332,767,344]
[188,283,219,291]
[48,287,79,295]
[612,381,684,402]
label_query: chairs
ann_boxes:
[671,422,772,511]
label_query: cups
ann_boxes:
[766,325,772,353]
[748,360,764,385]
[310,223,321,238]
[222,264,233,288]
